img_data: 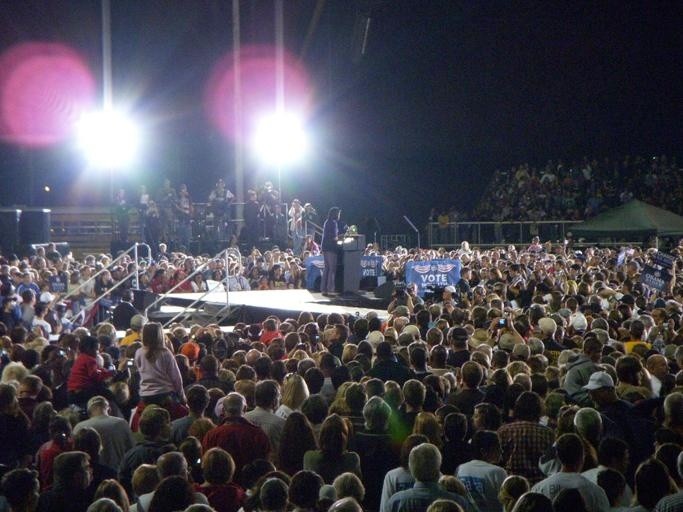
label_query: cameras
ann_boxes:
[127,359,134,367]
[499,319,507,326]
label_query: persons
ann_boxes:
[321,206,345,295]
[429,151,683,245]
[0,238,682,512]
[111,178,315,253]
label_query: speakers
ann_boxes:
[376,281,393,297]
[361,276,386,289]
[131,287,161,312]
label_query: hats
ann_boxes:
[569,312,587,331]
[40,292,56,303]
[580,371,613,391]
[538,317,556,334]
[467,328,495,348]
[86,396,109,409]
